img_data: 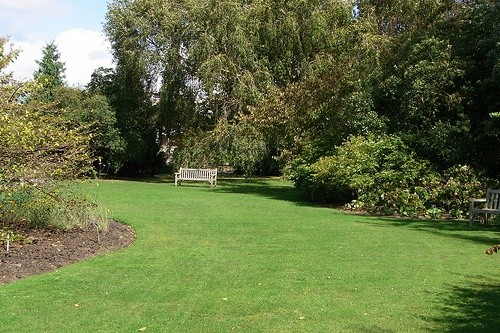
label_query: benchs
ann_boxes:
[468,187,500,227]
[174,166,218,188]
